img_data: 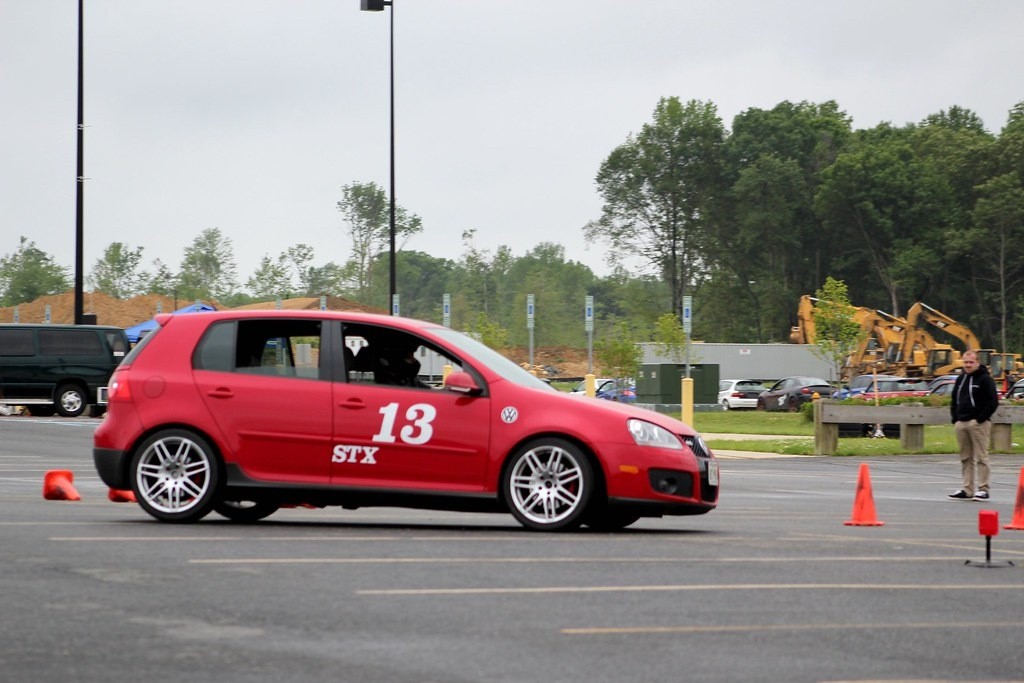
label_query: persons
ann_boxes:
[1002,370,1016,398]
[383,341,432,389]
[948,351,999,502]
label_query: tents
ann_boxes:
[123,303,217,344]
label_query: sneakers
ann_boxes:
[947,490,974,500]
[972,491,990,501]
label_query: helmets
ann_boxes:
[368,330,421,382]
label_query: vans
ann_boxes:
[0,322,134,418]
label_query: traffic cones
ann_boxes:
[1001,466,1024,531]
[41,468,81,501]
[107,487,137,504]
[842,462,886,526]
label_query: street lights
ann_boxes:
[360,0,400,315]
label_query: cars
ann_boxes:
[566,289,1024,405]
[89,305,722,534]
